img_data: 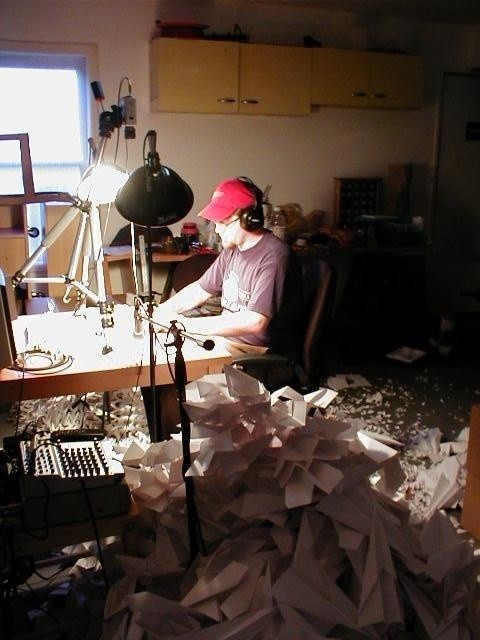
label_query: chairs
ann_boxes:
[254,258,334,380]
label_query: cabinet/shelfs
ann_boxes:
[313,49,422,110]
[149,37,310,115]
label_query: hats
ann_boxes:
[197,177,262,221]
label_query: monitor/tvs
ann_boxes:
[0,267,17,370]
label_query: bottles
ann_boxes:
[269,205,287,242]
[181,222,198,246]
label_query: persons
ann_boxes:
[152,178,296,379]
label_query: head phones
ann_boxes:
[237,176,264,232]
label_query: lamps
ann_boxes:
[61,159,128,354]
[114,130,195,442]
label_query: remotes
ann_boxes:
[50,429,105,443]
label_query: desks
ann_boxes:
[0,304,236,444]
[106,245,336,337]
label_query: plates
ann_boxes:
[155,20,211,30]
